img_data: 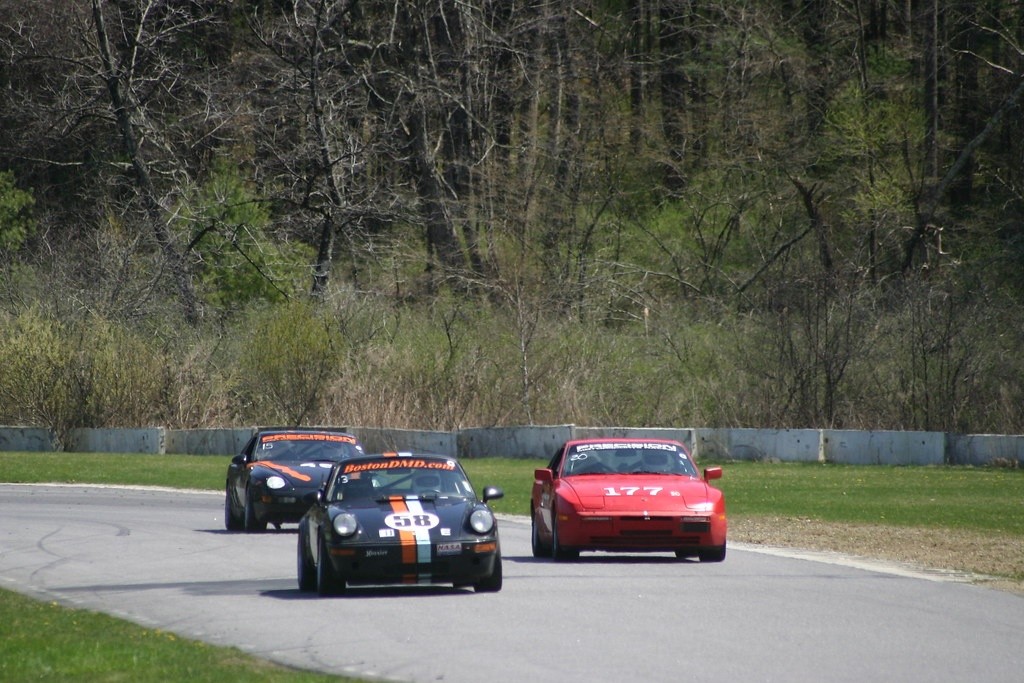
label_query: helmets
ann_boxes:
[641,449,675,471]
[412,468,441,493]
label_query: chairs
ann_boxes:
[642,454,673,474]
[342,478,375,501]
[572,456,605,475]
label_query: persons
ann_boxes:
[634,450,674,472]
[411,470,442,493]
[320,441,349,458]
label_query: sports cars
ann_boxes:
[224,429,378,534]
[297,453,505,598]
[530,437,728,563]
